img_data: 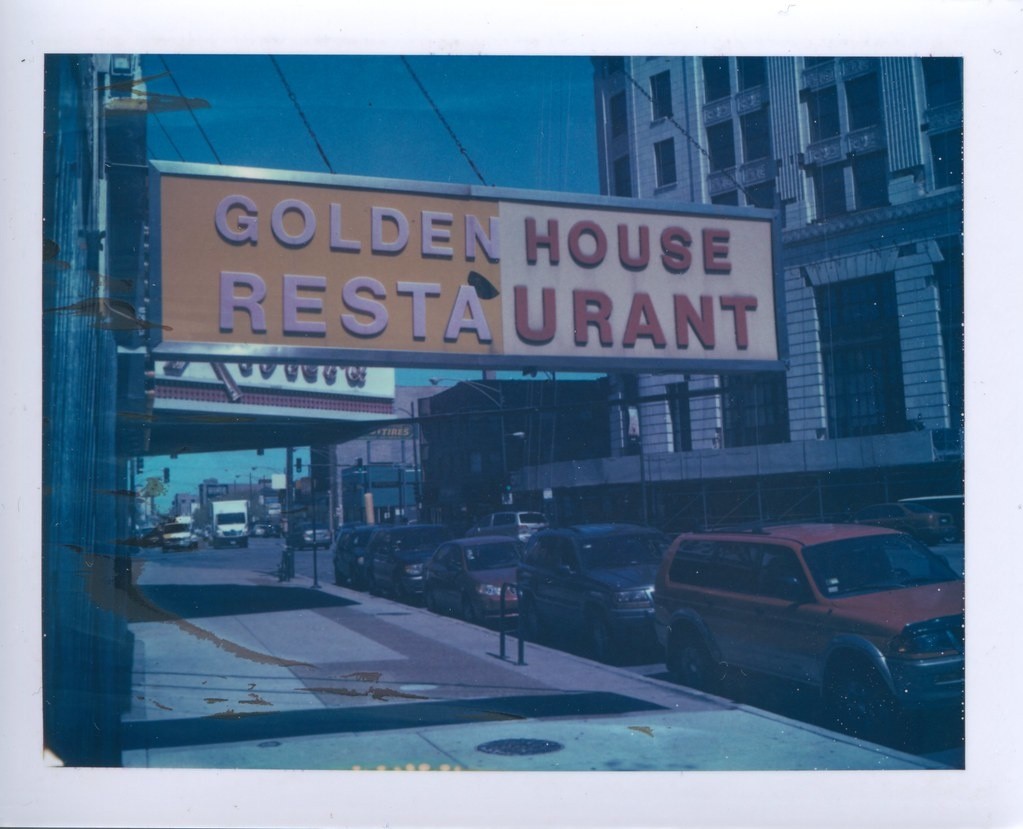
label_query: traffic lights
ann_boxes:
[163,468,170,484]
[356,457,363,472]
[136,457,144,474]
[502,471,513,495]
[296,458,303,473]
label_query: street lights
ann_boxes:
[427,374,512,510]
[235,472,253,517]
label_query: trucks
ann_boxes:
[208,499,251,549]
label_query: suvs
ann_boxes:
[467,510,550,544]
[331,522,400,589]
[365,522,456,607]
[653,519,965,744]
[515,518,679,667]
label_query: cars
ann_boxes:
[249,521,279,539]
[130,514,213,553]
[842,502,953,547]
[424,534,527,628]
[284,521,332,551]
[897,493,964,544]
